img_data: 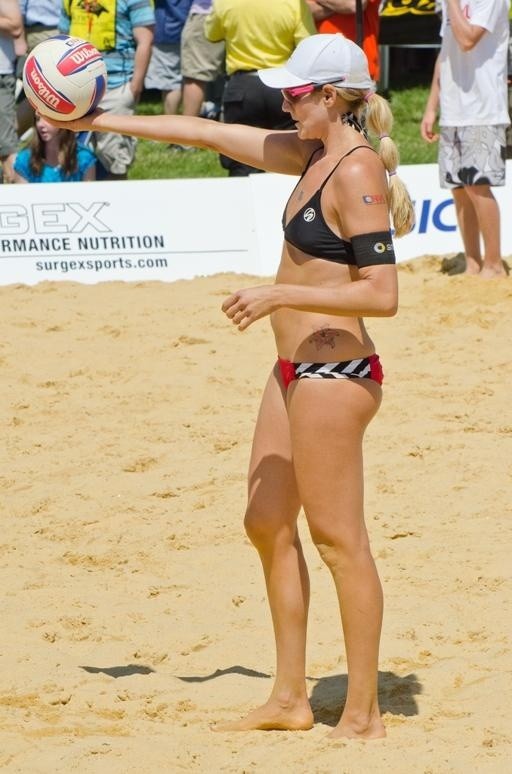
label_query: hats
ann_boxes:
[255,32,377,89]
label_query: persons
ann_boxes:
[419,0,512,280]
[28,31,418,744]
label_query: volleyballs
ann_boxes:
[23,35,107,122]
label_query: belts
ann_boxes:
[226,68,260,81]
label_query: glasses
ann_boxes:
[279,75,347,104]
